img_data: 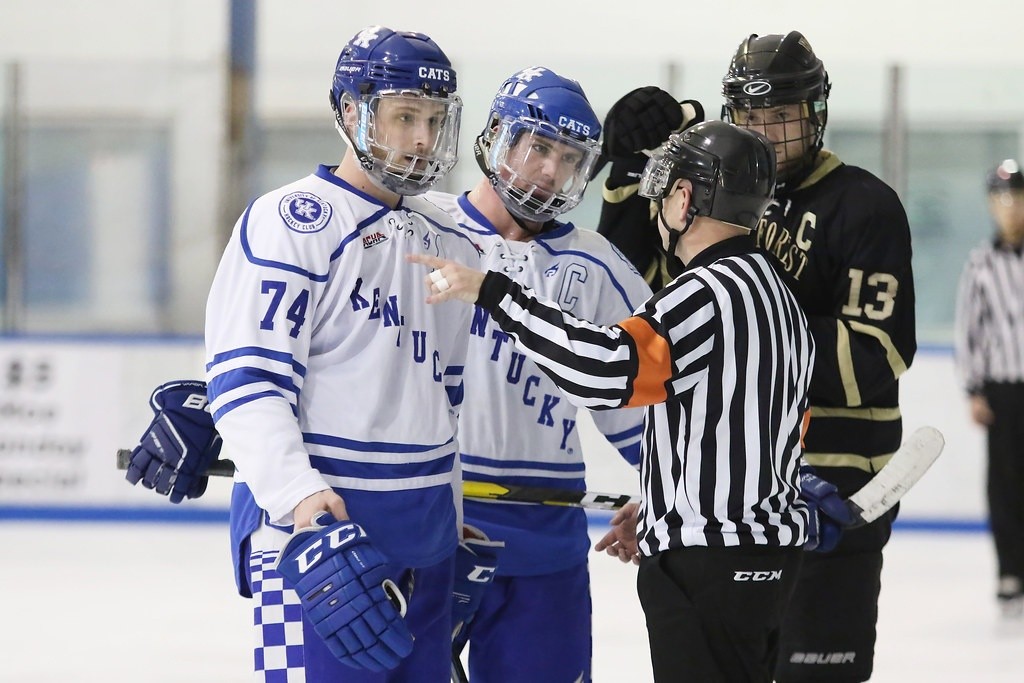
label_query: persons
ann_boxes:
[598,31,918,683]
[203,27,496,683]
[425,68,657,683]
[408,118,816,683]
[959,163,1024,598]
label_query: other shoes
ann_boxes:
[998,574,1024,600]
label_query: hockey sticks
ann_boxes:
[116,422,948,543]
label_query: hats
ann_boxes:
[988,166,1024,192]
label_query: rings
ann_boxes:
[436,278,450,292]
[429,269,442,283]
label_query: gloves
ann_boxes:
[800,472,858,559]
[603,86,689,165]
[273,510,415,672]
[126,379,223,504]
[451,522,505,642]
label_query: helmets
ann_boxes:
[638,120,777,230]
[329,24,463,195]
[473,66,602,222]
[721,30,830,194]
